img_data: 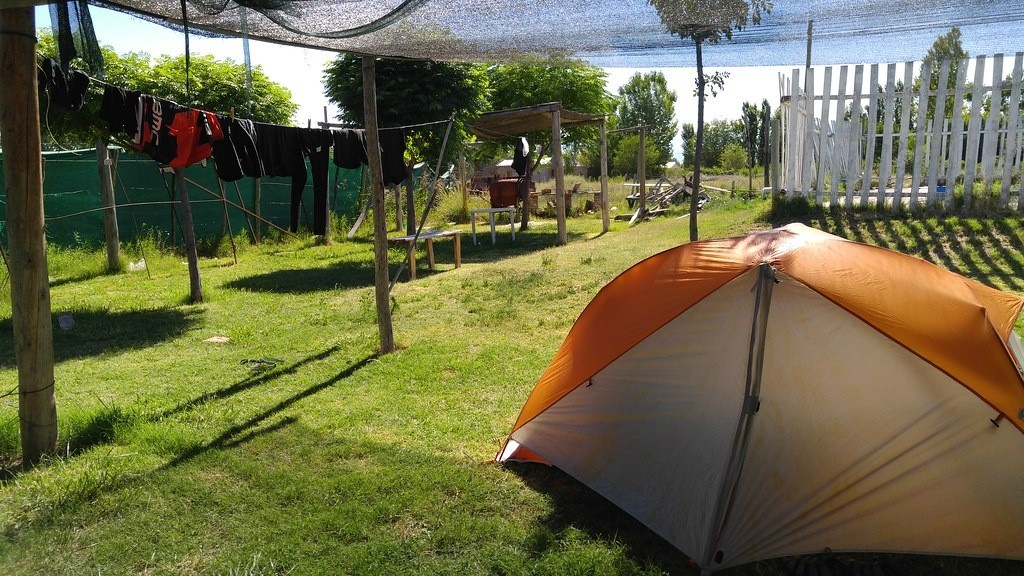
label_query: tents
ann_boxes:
[493,222,1023,576]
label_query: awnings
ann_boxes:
[1,0,1024,465]
[465,106,610,244]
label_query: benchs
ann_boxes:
[626,195,657,209]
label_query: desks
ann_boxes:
[469,208,515,246]
[528,190,602,219]
[623,183,672,194]
[369,230,462,282]
